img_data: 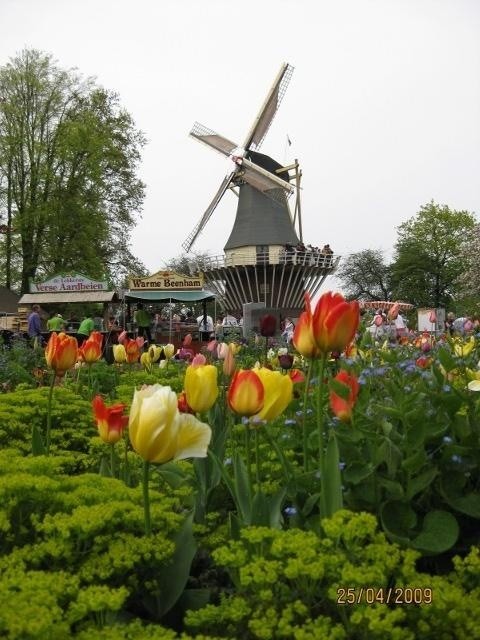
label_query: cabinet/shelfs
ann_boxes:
[7,317,19,330]
[17,306,32,330]
[129,321,200,343]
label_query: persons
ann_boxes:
[28,304,94,346]
[282,317,294,344]
[172,311,238,342]
[134,303,153,345]
[445,312,472,337]
[373,308,405,343]
